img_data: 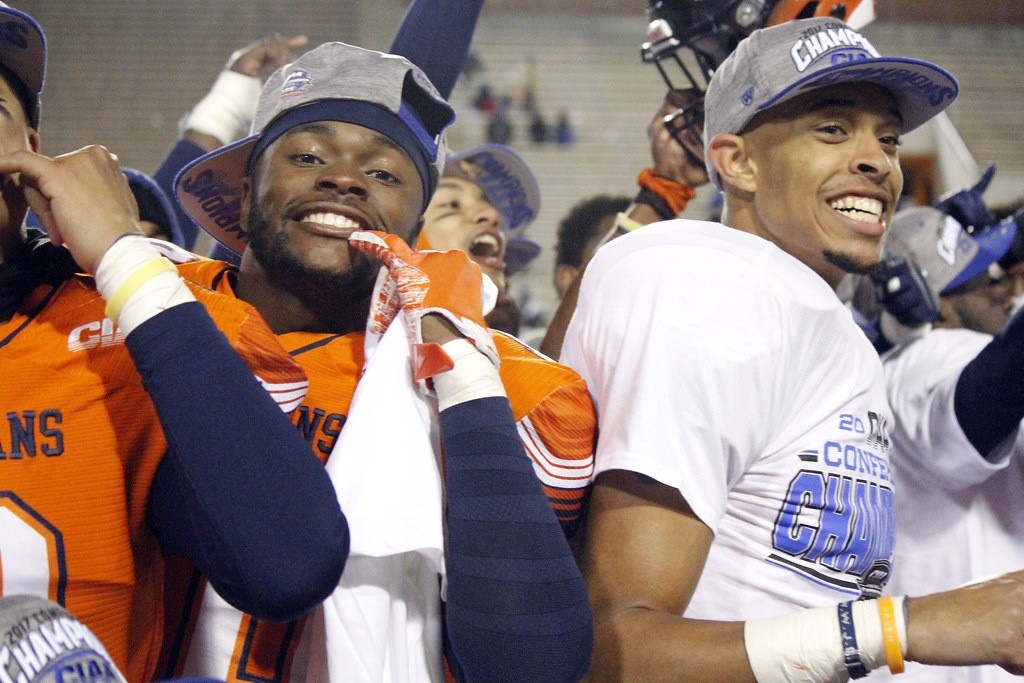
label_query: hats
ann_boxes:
[0,2,46,132]
[881,205,1018,298]
[504,238,542,278]
[443,143,542,239]
[171,42,458,259]
[23,167,180,244]
[703,16,962,194]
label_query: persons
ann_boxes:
[0,0,598,682]
[873,205,1024,683]
[537,16,1024,683]
[148,43,597,683]
[1,0,350,683]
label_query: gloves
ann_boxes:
[872,248,939,347]
[933,163,999,234]
[348,227,503,401]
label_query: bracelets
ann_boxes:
[103,256,180,324]
[615,212,645,231]
[630,186,676,221]
[838,600,867,680]
[877,595,904,674]
[637,169,693,215]
